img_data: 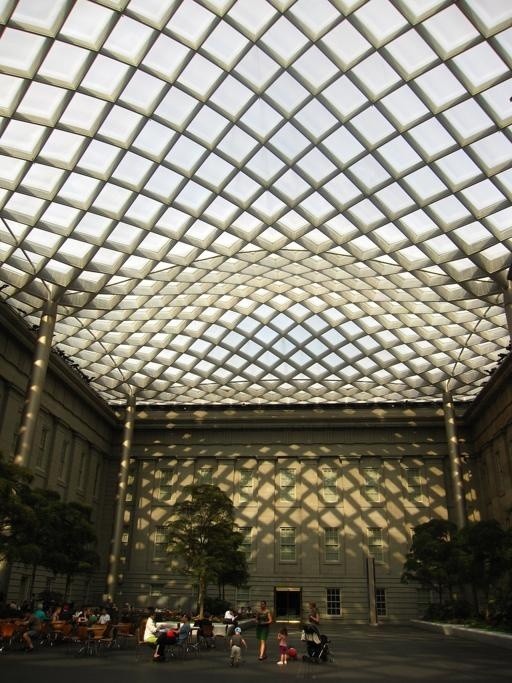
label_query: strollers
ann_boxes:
[299,624,335,663]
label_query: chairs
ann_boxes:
[0,613,212,662]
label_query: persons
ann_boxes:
[308,600,320,626]
[5,595,252,667]
[256,601,272,660]
[277,627,288,665]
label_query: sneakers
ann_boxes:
[258,655,288,665]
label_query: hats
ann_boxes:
[234,627,241,632]
